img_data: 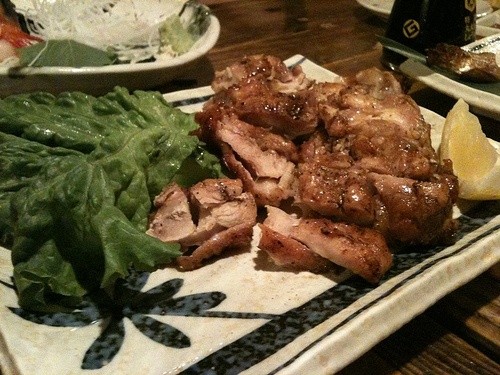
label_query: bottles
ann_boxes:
[381,0,477,71]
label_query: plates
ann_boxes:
[0,54,500,374]
[0,0,219,91]
[358,0,493,21]
[400,33,499,120]
[476,7,500,37]
